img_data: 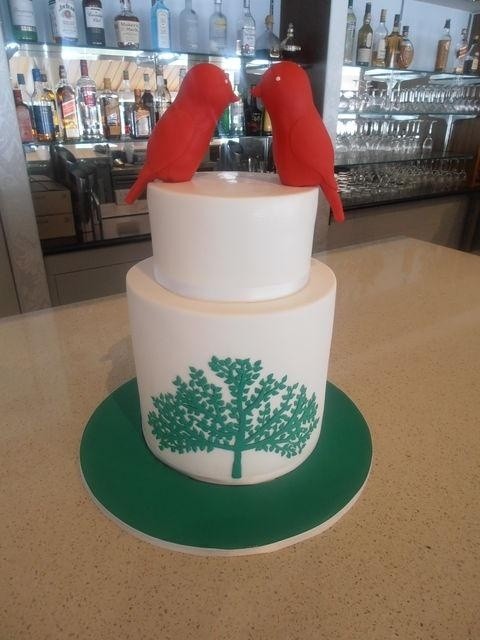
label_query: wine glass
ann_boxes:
[333,158,474,207]
[335,119,446,159]
[337,78,479,116]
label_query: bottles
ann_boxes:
[47,0,80,45]
[13,58,274,140]
[112,0,142,50]
[82,1,106,47]
[208,0,227,55]
[279,22,303,60]
[234,0,258,57]
[254,14,281,58]
[8,1,38,45]
[148,1,173,51]
[342,2,479,74]
[178,1,200,53]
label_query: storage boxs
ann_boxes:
[28,174,77,240]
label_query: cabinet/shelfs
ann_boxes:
[6,38,317,257]
[332,63,480,212]
